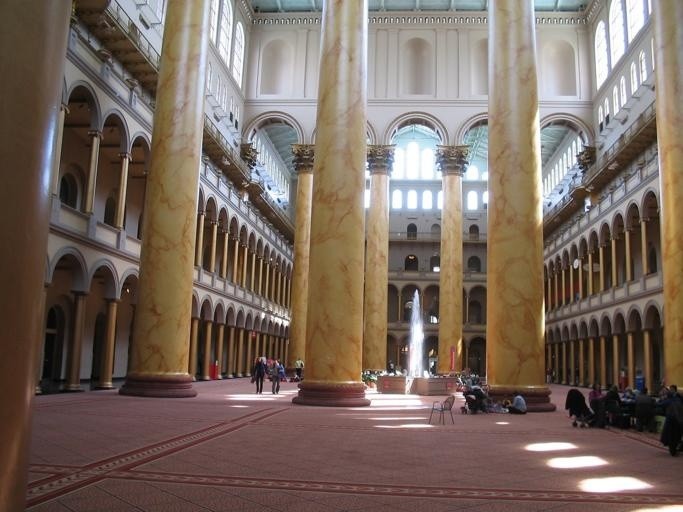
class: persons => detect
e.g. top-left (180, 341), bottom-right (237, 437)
top-left (252, 356), bottom-right (268, 394)
top-left (294, 358), bottom-right (304, 380)
top-left (506, 391), bottom-right (527, 414)
top-left (545, 368), bottom-right (552, 385)
top-left (269, 357), bottom-right (284, 393)
top-left (589, 380), bottom-right (683, 456)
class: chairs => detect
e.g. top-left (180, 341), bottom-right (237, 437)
top-left (429, 395), bottom-right (456, 426)
